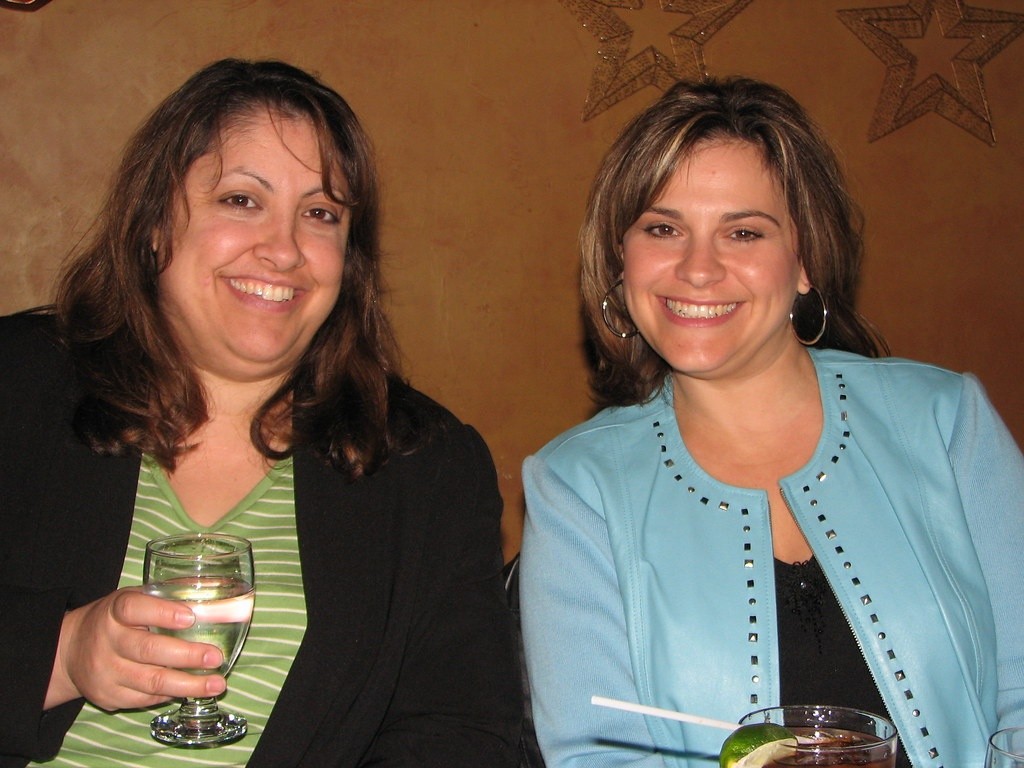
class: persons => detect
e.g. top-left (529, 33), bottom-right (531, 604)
top-left (2, 53), bottom-right (522, 768)
top-left (518, 74), bottom-right (1023, 768)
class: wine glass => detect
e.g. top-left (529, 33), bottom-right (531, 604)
top-left (143, 533), bottom-right (255, 747)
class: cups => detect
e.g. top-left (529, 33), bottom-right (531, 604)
top-left (984, 728), bottom-right (1024, 768)
top-left (739, 705), bottom-right (898, 768)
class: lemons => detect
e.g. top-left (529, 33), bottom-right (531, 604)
top-left (720, 723), bottom-right (798, 768)
